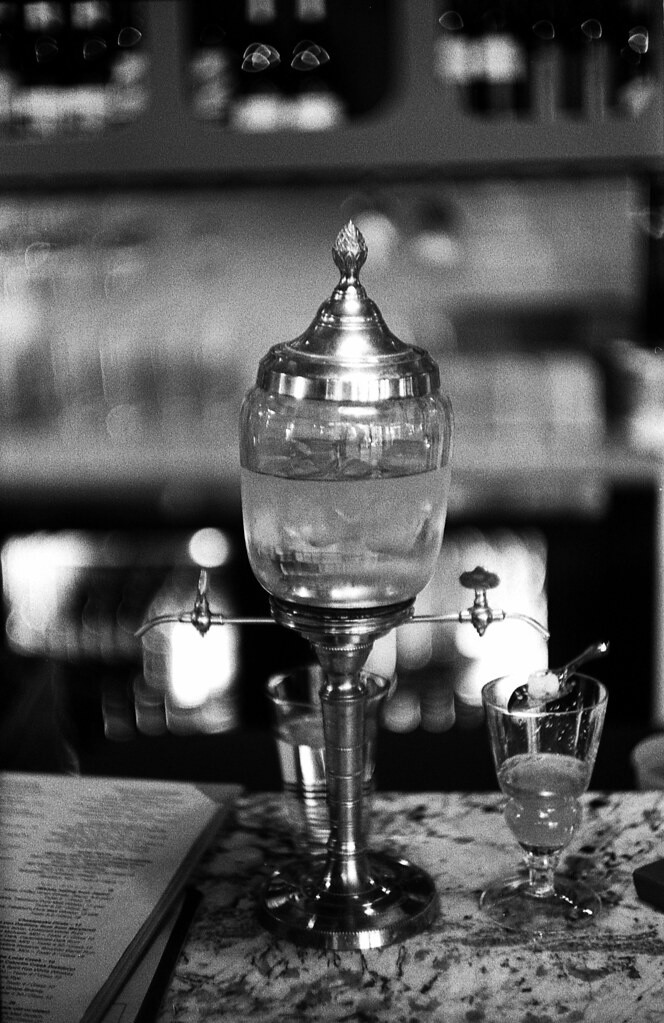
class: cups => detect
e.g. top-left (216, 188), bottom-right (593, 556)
top-left (632, 735), bottom-right (664, 858)
top-left (265, 664), bottom-right (391, 867)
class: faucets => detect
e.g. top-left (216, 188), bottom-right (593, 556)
top-left (456, 565), bottom-right (553, 641)
top-left (133, 564), bottom-right (224, 640)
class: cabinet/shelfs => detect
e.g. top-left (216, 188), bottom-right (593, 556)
top-left (1, 0), bottom-right (664, 182)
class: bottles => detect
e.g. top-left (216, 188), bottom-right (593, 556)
top-left (0, 1), bottom-right (664, 137)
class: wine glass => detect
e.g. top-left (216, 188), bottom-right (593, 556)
top-left (476, 667), bottom-right (610, 935)
